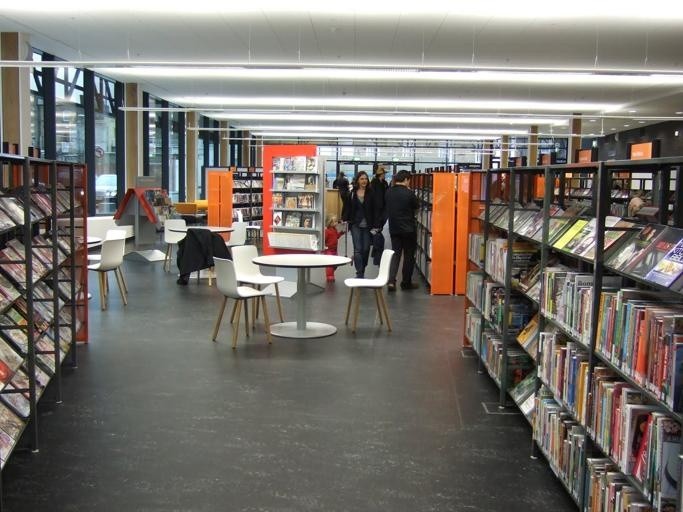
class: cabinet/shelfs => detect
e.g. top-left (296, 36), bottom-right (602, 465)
top-left (0, 148), bottom-right (89, 471)
top-left (270, 157), bottom-right (326, 248)
top-left (205, 171), bottom-right (262, 245)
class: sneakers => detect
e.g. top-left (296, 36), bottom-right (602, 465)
top-left (387, 281), bottom-right (420, 291)
top-left (371, 253), bottom-right (381, 265)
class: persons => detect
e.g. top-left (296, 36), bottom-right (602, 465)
top-left (332, 166), bottom-right (389, 267)
top-left (341, 171), bottom-right (382, 279)
top-left (319, 212), bottom-right (348, 282)
top-left (386, 169), bottom-right (420, 291)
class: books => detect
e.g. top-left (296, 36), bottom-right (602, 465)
top-left (232, 153), bottom-right (319, 238)
top-left (413, 174), bottom-right (431, 284)
top-left (462, 173), bottom-right (682, 512)
top-left (0, 180), bottom-right (82, 471)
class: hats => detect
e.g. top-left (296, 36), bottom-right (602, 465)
top-left (376, 167), bottom-right (387, 175)
